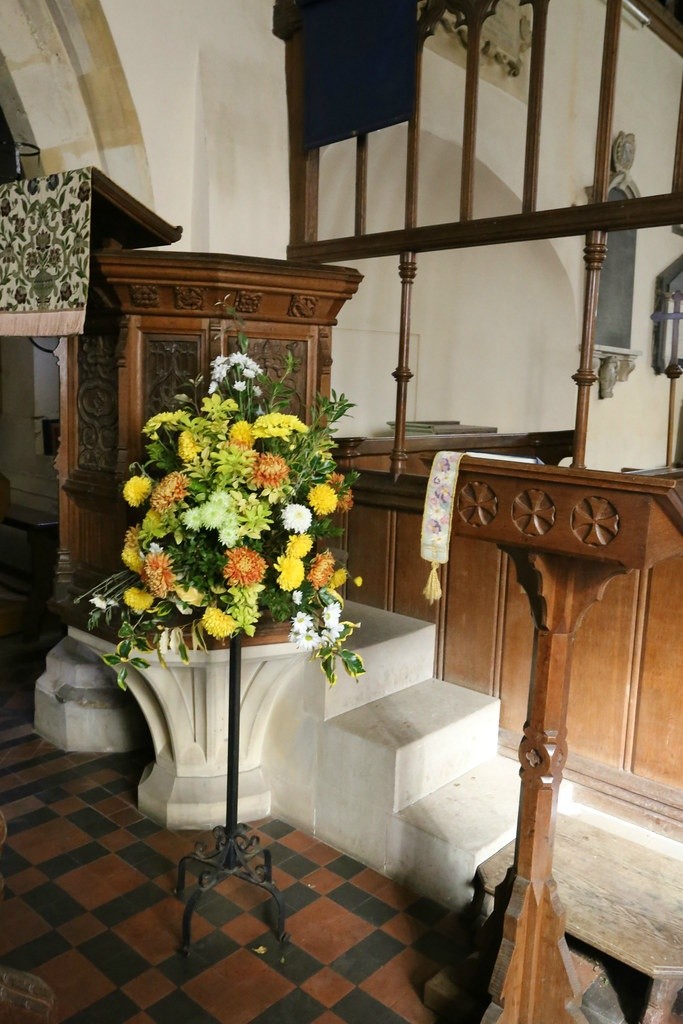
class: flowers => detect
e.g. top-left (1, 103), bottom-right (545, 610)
top-left (74, 293), bottom-right (367, 689)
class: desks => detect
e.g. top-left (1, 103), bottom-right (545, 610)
top-left (338, 429), bottom-right (578, 475)
